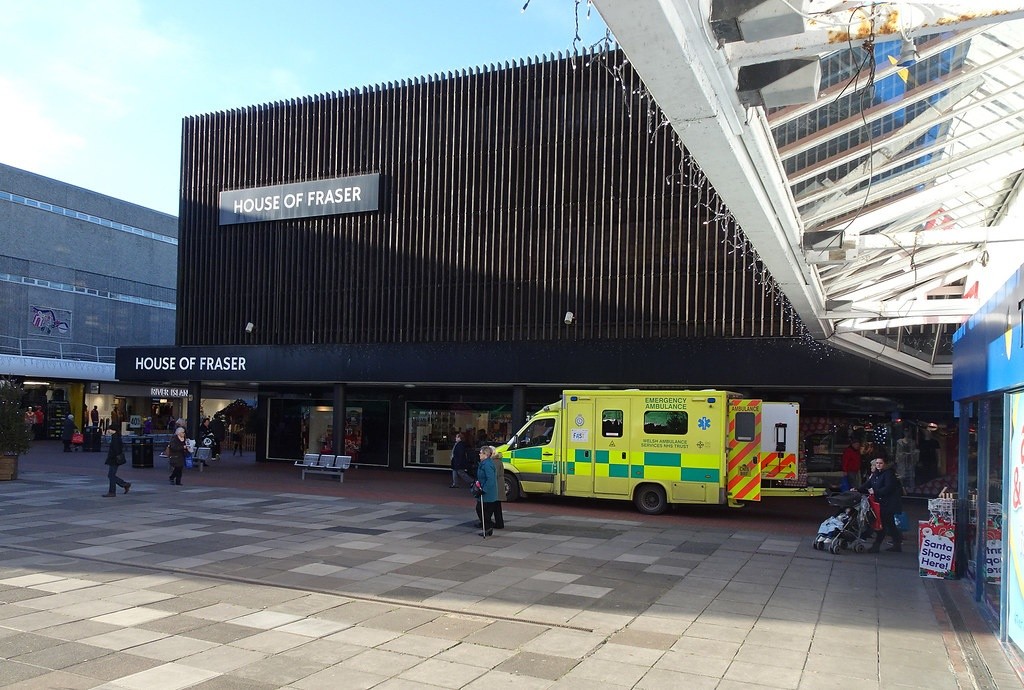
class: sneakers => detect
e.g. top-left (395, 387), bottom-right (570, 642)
top-left (817, 537), bottom-right (825, 541)
top-left (824, 538), bottom-right (832, 543)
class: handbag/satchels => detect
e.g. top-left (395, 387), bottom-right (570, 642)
top-left (869, 495), bottom-right (883, 531)
top-left (184, 451), bottom-right (193, 468)
top-left (71, 431), bottom-right (83, 444)
top-left (894, 511), bottom-right (911, 532)
top-left (115, 454), bottom-right (126, 465)
top-left (839, 476), bottom-right (849, 492)
top-left (470, 482), bottom-right (480, 497)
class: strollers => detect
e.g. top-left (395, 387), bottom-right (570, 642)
top-left (812, 491), bottom-right (872, 555)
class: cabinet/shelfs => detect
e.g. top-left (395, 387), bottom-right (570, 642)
top-left (411, 409), bottom-right (455, 463)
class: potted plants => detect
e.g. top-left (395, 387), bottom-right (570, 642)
top-left (0, 372), bottom-right (36, 481)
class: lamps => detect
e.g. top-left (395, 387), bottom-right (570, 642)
top-left (564, 312), bottom-right (576, 325)
top-left (245, 322), bottom-right (255, 333)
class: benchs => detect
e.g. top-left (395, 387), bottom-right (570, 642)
top-left (294, 454), bottom-right (352, 483)
top-left (99, 434), bottom-right (174, 452)
top-left (159, 445), bottom-right (212, 472)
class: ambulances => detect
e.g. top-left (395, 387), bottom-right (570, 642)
top-left (491, 388), bottom-right (801, 516)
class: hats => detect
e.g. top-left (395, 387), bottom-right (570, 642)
top-left (849, 435), bottom-right (861, 445)
top-left (175, 428), bottom-right (185, 434)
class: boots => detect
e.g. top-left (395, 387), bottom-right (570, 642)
top-left (169, 474), bottom-right (183, 486)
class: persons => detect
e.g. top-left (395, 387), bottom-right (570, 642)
top-left (25, 406), bottom-right (45, 441)
top-left (916, 430), bottom-right (937, 487)
top-left (100, 407), bottom-right (120, 434)
top-left (143, 417), bottom-right (152, 434)
top-left (167, 427), bottom-right (188, 485)
top-left (471, 445), bottom-right (507, 536)
top-left (199, 414), bottom-right (225, 466)
top-left (818, 436), bottom-right (903, 553)
top-left (84, 405), bottom-right (89, 427)
top-left (101, 423), bottom-right (132, 497)
top-left (62, 415), bottom-right (78, 452)
top-left (158, 416), bottom-right (187, 457)
top-left (895, 428), bottom-right (913, 495)
top-left (233, 423), bottom-right (246, 457)
top-left (90, 406), bottom-right (99, 432)
top-left (449, 427), bottom-right (494, 488)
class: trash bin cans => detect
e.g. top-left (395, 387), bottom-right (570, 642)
top-left (83, 426), bottom-right (102, 452)
top-left (131, 437), bottom-right (154, 468)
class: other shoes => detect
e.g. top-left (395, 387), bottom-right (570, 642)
top-left (102, 492), bottom-right (116, 497)
top-left (474, 521), bottom-right (503, 536)
top-left (123, 482), bottom-right (130, 494)
top-left (858, 531), bottom-right (903, 553)
